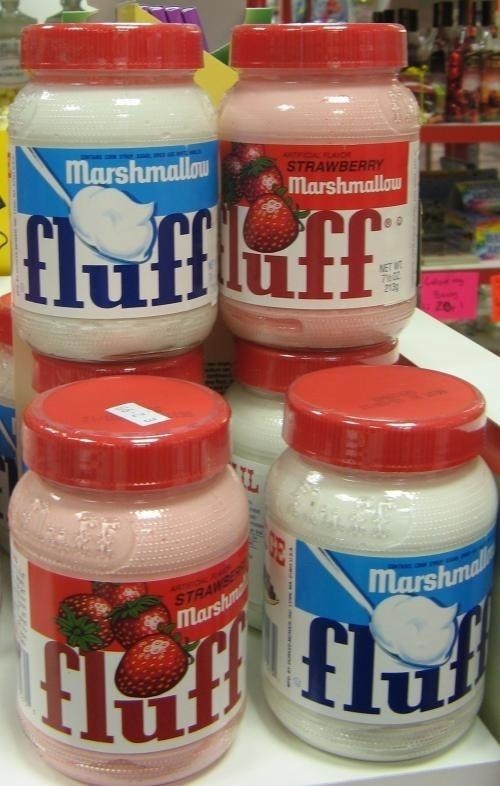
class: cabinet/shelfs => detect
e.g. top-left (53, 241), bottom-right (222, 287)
top-left (420, 122), bottom-right (500, 288)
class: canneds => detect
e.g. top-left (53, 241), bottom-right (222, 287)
top-left (262, 365), bottom-right (499, 762)
top-left (7, 373), bottom-right (249, 786)
top-left (6, 21), bottom-right (219, 362)
top-left (220, 24), bottom-right (424, 349)
top-left (2, 290), bottom-right (398, 632)
